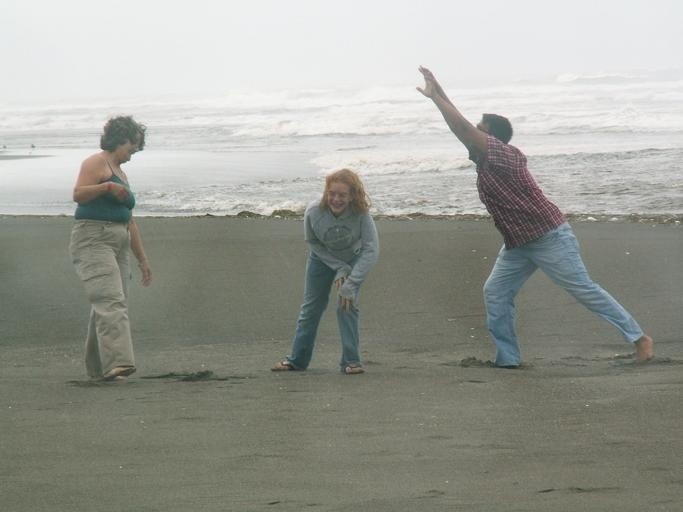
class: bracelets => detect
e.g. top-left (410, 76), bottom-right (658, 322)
top-left (107, 181), bottom-right (114, 194)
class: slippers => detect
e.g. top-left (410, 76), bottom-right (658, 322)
top-left (343, 364), bottom-right (365, 374)
top-left (271, 361), bottom-right (295, 371)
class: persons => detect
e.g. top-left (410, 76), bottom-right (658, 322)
top-left (64, 115), bottom-right (153, 383)
top-left (413, 64), bottom-right (654, 369)
top-left (267, 169), bottom-right (380, 375)
